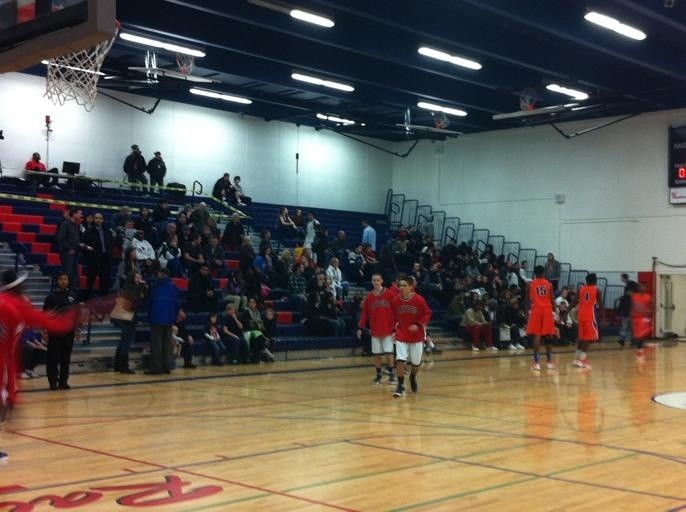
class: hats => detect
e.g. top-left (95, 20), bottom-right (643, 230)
top-left (0, 270), bottom-right (29, 292)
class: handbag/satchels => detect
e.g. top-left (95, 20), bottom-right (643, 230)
top-left (110, 297), bottom-right (135, 322)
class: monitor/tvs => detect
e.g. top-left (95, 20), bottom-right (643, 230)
top-left (62, 161), bottom-right (80, 176)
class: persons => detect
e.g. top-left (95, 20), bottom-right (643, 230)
top-left (212, 173), bottom-right (246, 206)
top-left (123, 144), bottom-right (150, 198)
top-left (26, 153), bottom-right (60, 189)
top-left (22, 323), bottom-right (48, 378)
top-left (232, 175), bottom-right (244, 195)
top-left (525, 265), bottom-right (555, 369)
top-left (223, 207), bottom-right (443, 354)
top-left (41, 274), bottom-right (74, 390)
top-left (147, 152), bottom-right (167, 197)
top-left (361, 219), bottom-right (584, 351)
top-left (0, 271), bottom-right (77, 462)
top-left (617, 273), bottom-right (641, 345)
top-left (631, 280), bottom-right (655, 361)
top-left (54, 204), bottom-right (277, 375)
top-left (564, 273), bottom-right (602, 367)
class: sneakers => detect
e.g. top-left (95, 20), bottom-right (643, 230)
top-left (361, 348), bottom-right (442, 397)
top-left (472, 340), bottom-right (656, 370)
